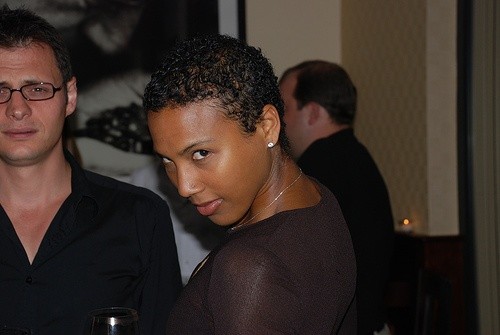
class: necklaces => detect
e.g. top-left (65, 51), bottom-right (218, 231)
top-left (231, 167), bottom-right (303, 230)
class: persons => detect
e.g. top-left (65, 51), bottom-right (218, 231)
top-left (0, 7), bottom-right (184, 335)
top-left (142, 32), bottom-right (357, 335)
top-left (278, 60), bottom-right (395, 335)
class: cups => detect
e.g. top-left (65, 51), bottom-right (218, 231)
top-left (86, 307), bottom-right (139, 335)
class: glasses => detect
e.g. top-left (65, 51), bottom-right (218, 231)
top-left (0, 82), bottom-right (63, 104)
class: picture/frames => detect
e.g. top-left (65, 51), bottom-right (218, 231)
top-left (0, 0), bottom-right (246, 287)
